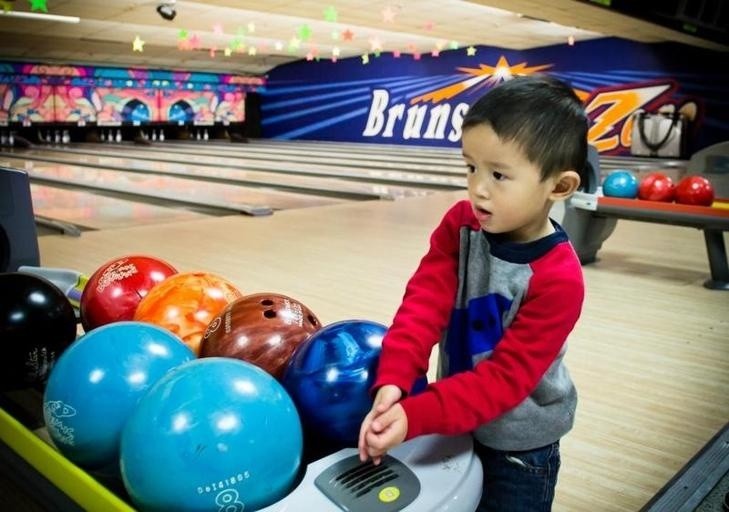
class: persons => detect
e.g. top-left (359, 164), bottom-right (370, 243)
top-left (358, 74), bottom-right (588, 512)
